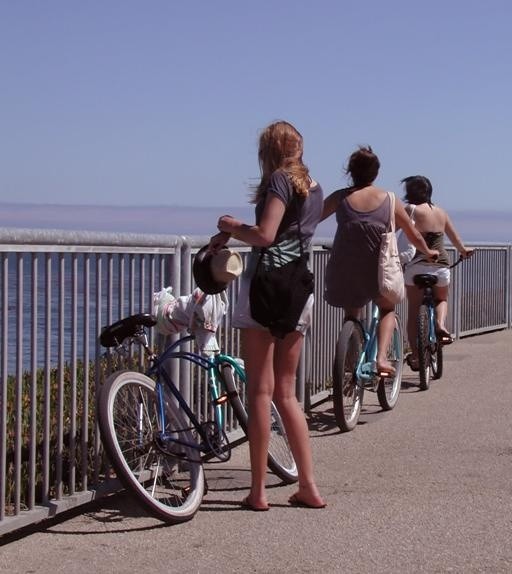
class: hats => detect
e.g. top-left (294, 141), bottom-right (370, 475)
top-left (192, 241), bottom-right (243, 296)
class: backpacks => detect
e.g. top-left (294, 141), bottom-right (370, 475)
top-left (248, 246), bottom-right (315, 339)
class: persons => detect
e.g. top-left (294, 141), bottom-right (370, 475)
top-left (206, 121), bottom-right (325, 510)
top-left (391, 174), bottom-right (473, 372)
top-left (319, 144), bottom-right (440, 398)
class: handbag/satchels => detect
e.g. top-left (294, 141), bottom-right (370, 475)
top-left (392, 203), bottom-right (417, 262)
top-left (371, 190), bottom-right (406, 303)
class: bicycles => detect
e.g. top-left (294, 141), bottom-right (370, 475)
top-left (321, 244), bottom-right (438, 432)
top-left (97, 241), bottom-right (300, 526)
top-left (406, 249), bottom-right (476, 391)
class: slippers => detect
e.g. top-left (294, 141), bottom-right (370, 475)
top-left (288, 492), bottom-right (327, 512)
top-left (241, 493), bottom-right (270, 513)
